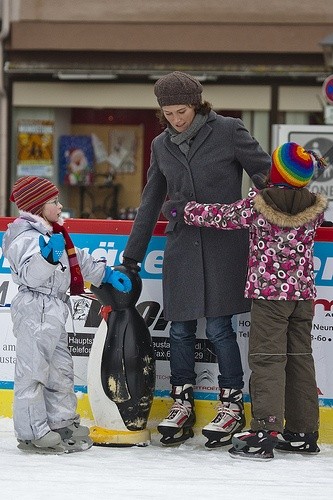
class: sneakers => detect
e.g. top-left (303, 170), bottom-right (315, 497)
top-left (53, 422), bottom-right (93, 451)
top-left (275, 428), bottom-right (320, 454)
top-left (156, 384), bottom-right (196, 446)
top-left (202, 388), bottom-right (246, 448)
top-left (228, 429), bottom-right (277, 462)
top-left (17, 430), bottom-right (65, 454)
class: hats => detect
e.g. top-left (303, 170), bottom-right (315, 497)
top-left (268, 142), bottom-right (327, 189)
top-left (9, 176), bottom-right (59, 215)
top-left (154, 71), bottom-right (203, 105)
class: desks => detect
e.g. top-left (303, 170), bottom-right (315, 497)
top-left (79, 183), bottom-right (123, 220)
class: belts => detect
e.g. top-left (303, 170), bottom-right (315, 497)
top-left (18, 284), bottom-right (76, 336)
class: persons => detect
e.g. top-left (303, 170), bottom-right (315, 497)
top-left (2, 175), bottom-right (133, 455)
top-left (121, 71), bottom-right (272, 448)
top-left (161, 143), bottom-right (330, 462)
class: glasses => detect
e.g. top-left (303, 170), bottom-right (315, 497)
top-left (45, 197), bottom-right (60, 206)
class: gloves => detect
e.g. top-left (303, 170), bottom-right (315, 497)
top-left (102, 265), bottom-right (132, 293)
top-left (161, 192), bottom-right (187, 223)
top-left (121, 256), bottom-right (141, 274)
top-left (39, 234), bottom-right (65, 265)
